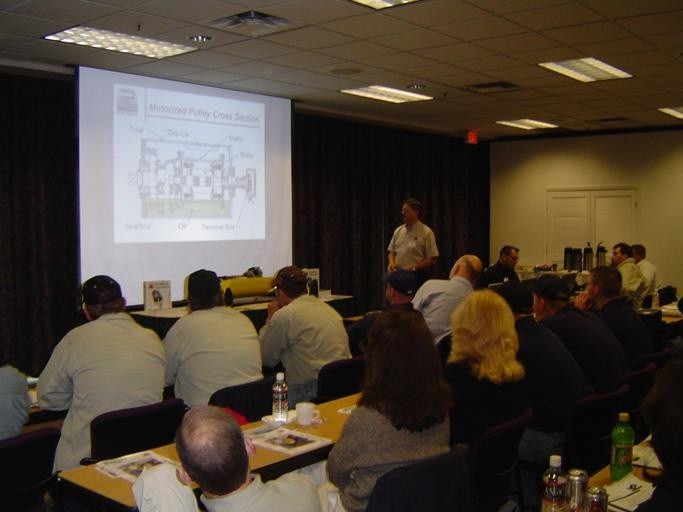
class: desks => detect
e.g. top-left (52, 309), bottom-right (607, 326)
top-left (130, 293), bottom-right (357, 340)
top-left (2, 375), bottom-right (67, 437)
top-left (59, 391), bottom-right (363, 511)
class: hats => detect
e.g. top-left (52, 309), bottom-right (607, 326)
top-left (525, 273), bottom-right (571, 302)
top-left (82, 274), bottom-right (122, 305)
top-left (380, 269), bottom-right (418, 296)
top-left (189, 269), bottom-right (220, 297)
top-left (270, 266), bottom-right (307, 287)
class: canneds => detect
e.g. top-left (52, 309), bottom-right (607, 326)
top-left (583, 485), bottom-right (607, 511)
top-left (565, 468), bottom-right (589, 511)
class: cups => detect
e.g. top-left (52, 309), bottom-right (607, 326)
top-left (296, 403), bottom-right (319, 426)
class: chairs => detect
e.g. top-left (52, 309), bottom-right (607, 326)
top-left (515, 267), bottom-right (591, 319)
top-left (633, 348), bottom-right (672, 369)
top-left (365, 439), bottom-right (508, 512)
top-left (207, 373), bottom-right (283, 422)
top-left (622, 363), bottom-right (660, 410)
top-left (1, 470), bottom-right (61, 511)
top-left (315, 358), bottom-right (365, 404)
top-left (476, 407), bottom-right (536, 510)
top-left (79, 397), bottom-right (190, 468)
top-left (566, 384), bottom-right (632, 468)
top-left (636, 285), bottom-right (682, 325)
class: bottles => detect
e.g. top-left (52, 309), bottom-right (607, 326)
top-left (653, 289), bottom-right (659, 308)
top-left (564, 246), bottom-right (608, 272)
top-left (272, 372), bottom-right (288, 420)
top-left (541, 455), bottom-right (567, 511)
top-left (610, 412), bottom-right (634, 484)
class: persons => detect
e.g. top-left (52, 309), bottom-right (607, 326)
top-left (387, 199), bottom-right (439, 285)
top-left (1, 244), bottom-right (683, 511)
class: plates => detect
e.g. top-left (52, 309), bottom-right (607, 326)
top-left (262, 414), bottom-right (293, 425)
